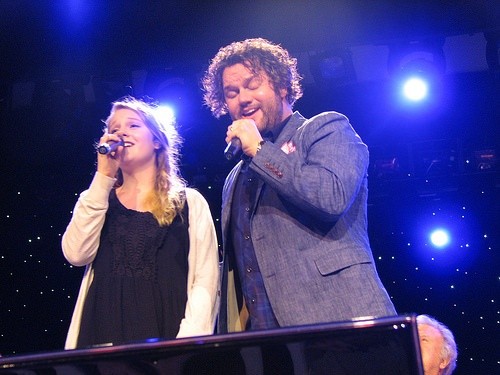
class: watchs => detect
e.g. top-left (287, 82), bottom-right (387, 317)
top-left (256, 141), bottom-right (268, 153)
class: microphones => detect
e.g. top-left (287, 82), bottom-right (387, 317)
top-left (224, 136), bottom-right (242, 160)
top-left (98, 137), bottom-right (124, 154)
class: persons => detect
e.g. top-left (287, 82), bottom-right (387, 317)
top-left (413, 315), bottom-right (458, 375)
top-left (60, 100), bottom-right (223, 355)
top-left (200, 38), bottom-right (397, 334)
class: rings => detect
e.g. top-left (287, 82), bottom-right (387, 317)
top-left (231, 126), bottom-right (233, 131)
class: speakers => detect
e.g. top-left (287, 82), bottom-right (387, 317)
top-left (0, 313), bottom-right (423, 375)
